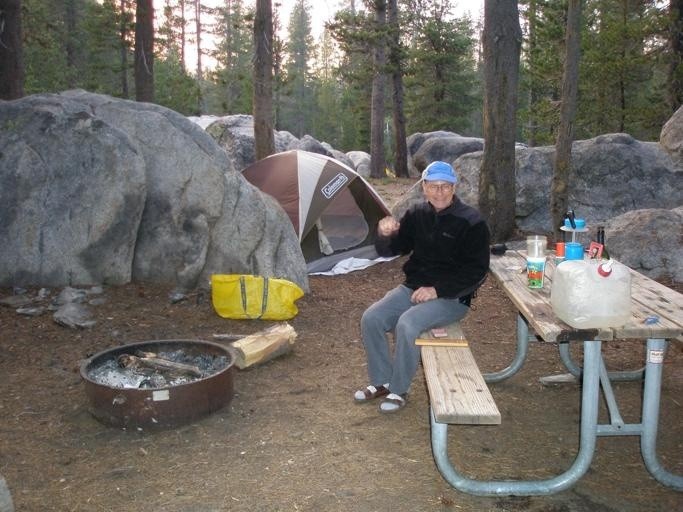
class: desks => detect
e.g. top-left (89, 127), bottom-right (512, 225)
top-left (489, 251), bottom-right (683, 494)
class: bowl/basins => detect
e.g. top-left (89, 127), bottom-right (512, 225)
top-left (78, 338), bottom-right (237, 432)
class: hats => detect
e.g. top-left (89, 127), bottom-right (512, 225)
top-left (421, 160), bottom-right (458, 183)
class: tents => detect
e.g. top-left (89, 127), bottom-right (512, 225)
top-left (240, 150), bottom-right (391, 276)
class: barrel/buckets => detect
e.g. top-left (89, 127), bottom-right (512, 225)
top-left (551, 258), bottom-right (633, 330)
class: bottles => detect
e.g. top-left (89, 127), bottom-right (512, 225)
top-left (591, 225), bottom-right (608, 260)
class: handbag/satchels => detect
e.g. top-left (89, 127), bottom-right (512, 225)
top-left (210, 273), bottom-right (305, 320)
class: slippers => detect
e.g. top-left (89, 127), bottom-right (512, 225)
top-left (354, 383), bottom-right (409, 413)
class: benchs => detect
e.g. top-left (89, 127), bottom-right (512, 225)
top-left (420, 319), bottom-right (502, 496)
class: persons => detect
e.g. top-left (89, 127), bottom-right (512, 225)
top-left (353, 160), bottom-right (491, 414)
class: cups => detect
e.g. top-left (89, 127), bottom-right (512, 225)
top-left (526, 256), bottom-right (547, 288)
top-left (564, 218), bottom-right (586, 260)
top-left (528, 235), bottom-right (547, 258)
top-left (556, 242), bottom-right (565, 257)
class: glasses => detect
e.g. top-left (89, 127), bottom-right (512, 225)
top-left (430, 184), bottom-right (449, 191)
